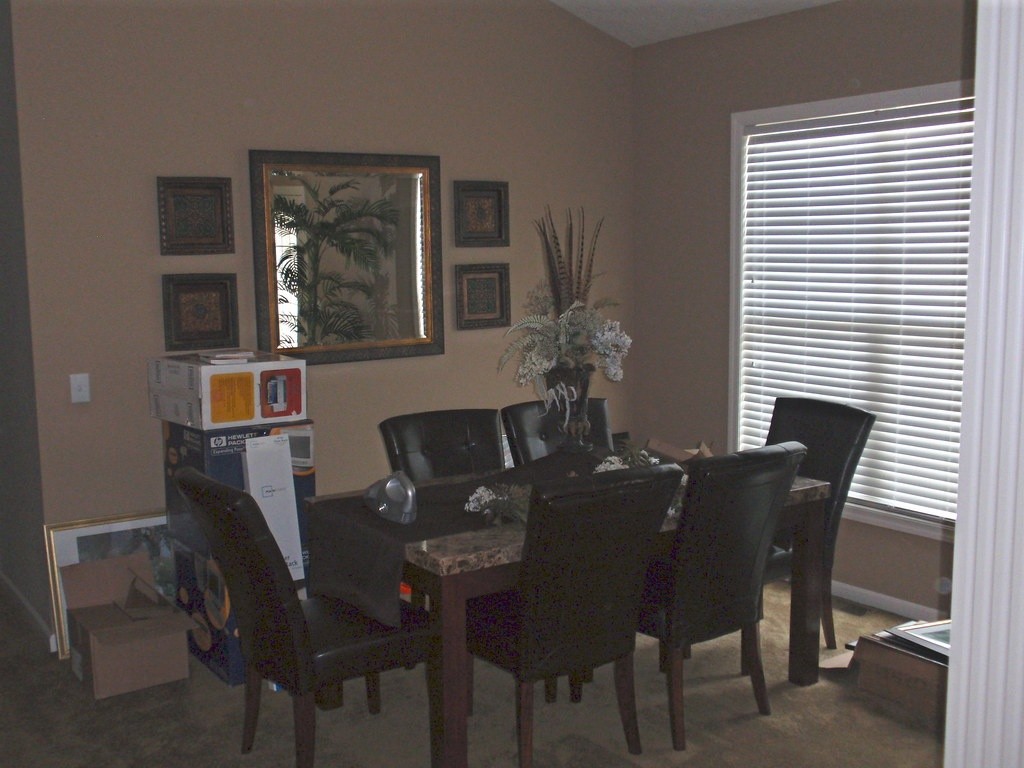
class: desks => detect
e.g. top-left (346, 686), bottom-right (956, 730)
top-left (300, 458), bottom-right (835, 768)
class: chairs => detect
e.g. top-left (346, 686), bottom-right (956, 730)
top-left (501, 391), bottom-right (613, 469)
top-left (642, 439), bottom-right (811, 751)
top-left (469, 460), bottom-right (683, 768)
top-left (744, 395), bottom-right (879, 672)
top-left (173, 462), bottom-right (430, 768)
top-left (374, 405), bottom-right (511, 480)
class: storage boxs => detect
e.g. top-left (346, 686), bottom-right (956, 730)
top-left (157, 427), bottom-right (320, 681)
top-left (148, 346), bottom-right (311, 426)
top-left (842, 620), bottom-right (947, 733)
top-left (69, 564), bottom-right (191, 701)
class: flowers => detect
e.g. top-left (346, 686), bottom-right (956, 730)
top-left (497, 198), bottom-right (636, 424)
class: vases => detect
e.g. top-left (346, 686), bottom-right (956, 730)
top-left (544, 363), bottom-right (598, 466)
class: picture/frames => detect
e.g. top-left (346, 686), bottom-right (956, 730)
top-left (153, 174), bottom-right (239, 257)
top-left (452, 178), bottom-right (512, 249)
top-left (454, 262), bottom-right (512, 332)
top-left (245, 148), bottom-right (447, 368)
top-left (161, 271), bottom-right (241, 351)
